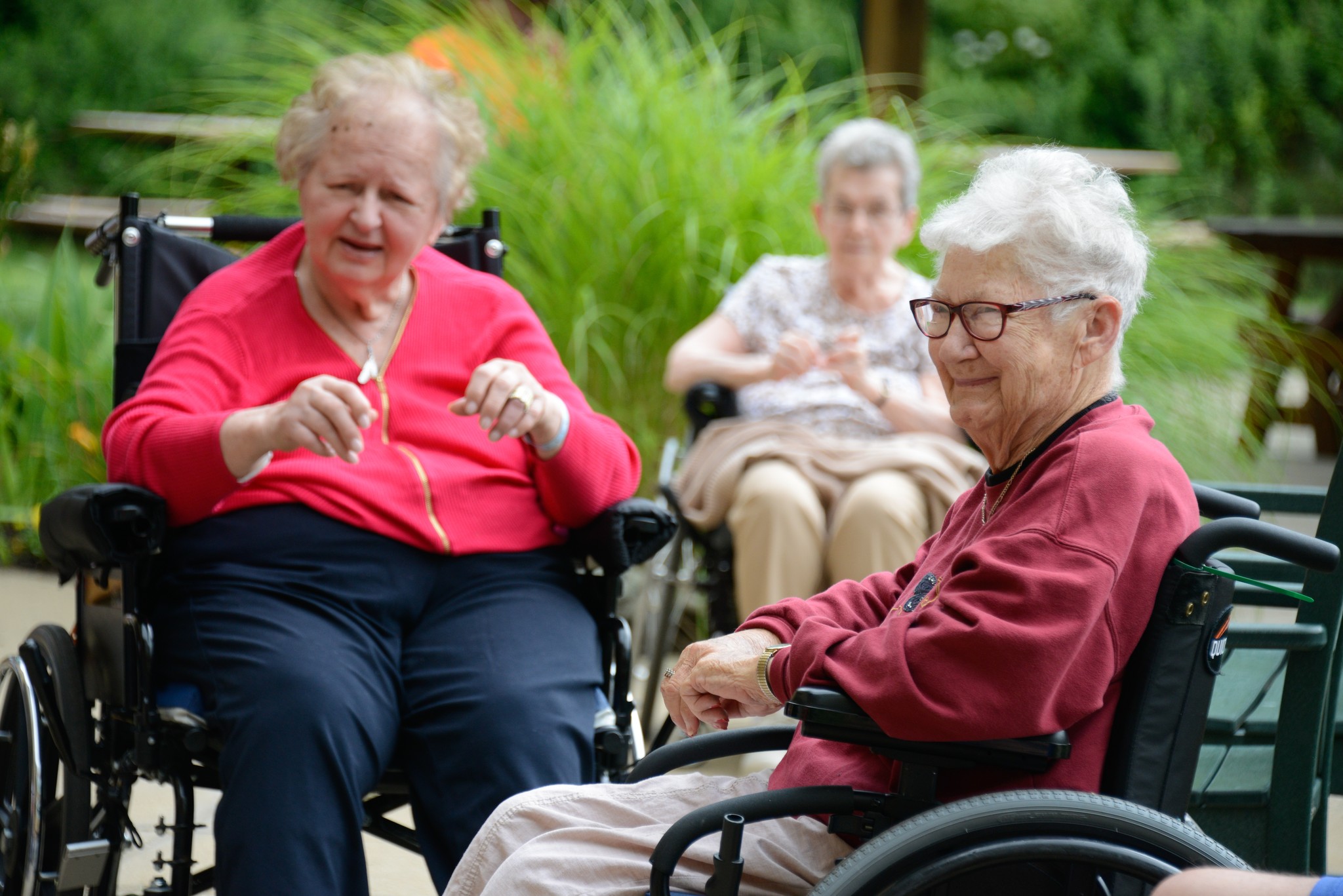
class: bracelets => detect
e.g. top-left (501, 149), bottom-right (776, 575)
top-left (523, 394), bottom-right (570, 452)
top-left (877, 383), bottom-right (892, 409)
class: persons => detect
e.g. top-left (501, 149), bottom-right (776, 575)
top-left (439, 146), bottom-right (1343, 896)
top-left (101, 52), bottom-right (644, 895)
top-left (661, 117), bottom-right (970, 623)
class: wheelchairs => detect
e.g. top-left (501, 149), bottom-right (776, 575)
top-left (622, 449), bottom-right (1343, 896)
top-left (0, 191), bottom-right (679, 896)
top-left (635, 383), bottom-right (740, 758)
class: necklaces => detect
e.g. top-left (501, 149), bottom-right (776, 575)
top-left (980, 449), bottom-right (1037, 527)
top-left (311, 274), bottom-right (403, 386)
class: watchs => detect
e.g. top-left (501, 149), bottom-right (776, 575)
top-left (756, 644), bottom-right (792, 706)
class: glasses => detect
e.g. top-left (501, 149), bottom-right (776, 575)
top-left (909, 293), bottom-right (1098, 339)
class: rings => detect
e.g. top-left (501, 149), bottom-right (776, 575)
top-left (664, 669), bottom-right (675, 679)
top-left (510, 384), bottom-right (534, 412)
